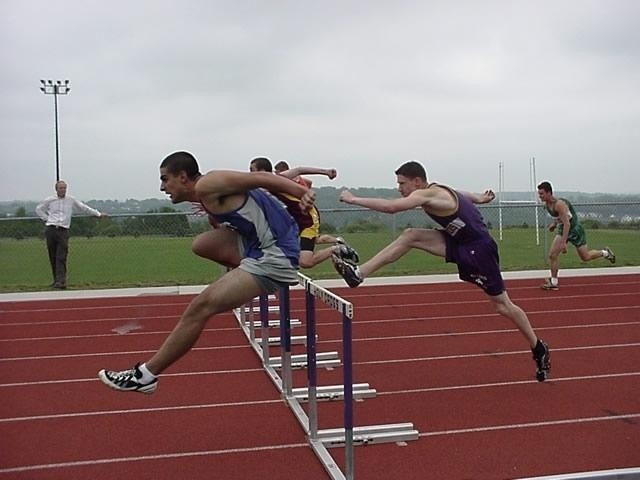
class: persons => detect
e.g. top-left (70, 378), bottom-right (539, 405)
top-left (330, 160), bottom-right (552, 383)
top-left (537, 181), bottom-right (616, 291)
top-left (99, 152), bottom-right (315, 394)
top-left (191, 158), bottom-right (359, 268)
top-left (275, 160), bottom-right (348, 245)
top-left (34, 180), bottom-right (107, 289)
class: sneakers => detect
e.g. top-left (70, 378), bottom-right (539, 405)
top-left (97, 364), bottom-right (157, 395)
top-left (532, 341), bottom-right (550, 383)
top-left (331, 252), bottom-right (363, 289)
top-left (541, 281), bottom-right (559, 291)
top-left (335, 236), bottom-right (359, 264)
top-left (603, 246), bottom-right (616, 264)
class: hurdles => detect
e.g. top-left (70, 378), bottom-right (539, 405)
top-left (233, 273), bottom-right (419, 480)
